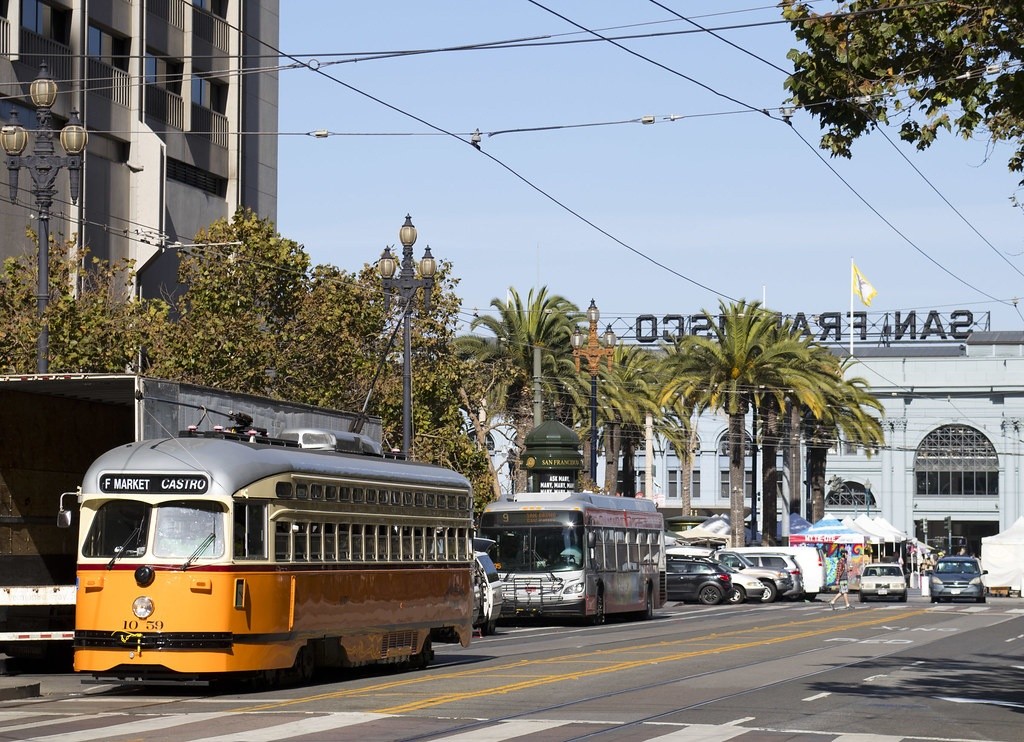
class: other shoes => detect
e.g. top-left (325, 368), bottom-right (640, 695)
top-left (847, 605), bottom-right (855, 610)
top-left (829, 602), bottom-right (835, 609)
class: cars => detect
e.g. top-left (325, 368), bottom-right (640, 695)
top-left (743, 552), bottom-right (805, 601)
top-left (856, 561), bottom-right (908, 603)
top-left (472, 549), bottom-right (503, 634)
top-left (703, 558), bottom-right (765, 605)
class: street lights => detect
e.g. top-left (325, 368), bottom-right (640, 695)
top-left (570, 296), bottom-right (618, 489)
top-left (863, 478), bottom-right (873, 517)
top-left (378, 216), bottom-right (437, 459)
top-left (2, 58), bottom-right (87, 373)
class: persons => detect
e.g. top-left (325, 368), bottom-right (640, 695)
top-left (828, 550), bottom-right (856, 610)
top-left (956, 547), bottom-right (971, 557)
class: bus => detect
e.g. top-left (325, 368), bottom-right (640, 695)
top-left (58, 412), bottom-right (474, 692)
top-left (479, 491), bottom-right (669, 626)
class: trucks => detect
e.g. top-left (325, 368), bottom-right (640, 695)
top-left (715, 546), bottom-right (827, 601)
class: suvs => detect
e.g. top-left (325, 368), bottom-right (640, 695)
top-left (711, 551), bottom-right (794, 603)
top-left (664, 555), bottom-right (736, 606)
top-left (926, 555), bottom-right (989, 604)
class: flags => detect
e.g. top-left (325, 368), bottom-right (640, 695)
top-left (851, 263), bottom-right (877, 306)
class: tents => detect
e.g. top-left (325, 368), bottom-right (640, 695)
top-left (685, 510), bottom-right (933, 588)
top-left (980, 515), bottom-right (1023, 597)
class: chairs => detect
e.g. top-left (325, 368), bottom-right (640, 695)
top-left (869, 570), bottom-right (877, 576)
top-left (888, 568), bottom-right (896, 576)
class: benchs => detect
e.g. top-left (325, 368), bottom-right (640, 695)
top-left (245, 533), bottom-right (466, 561)
